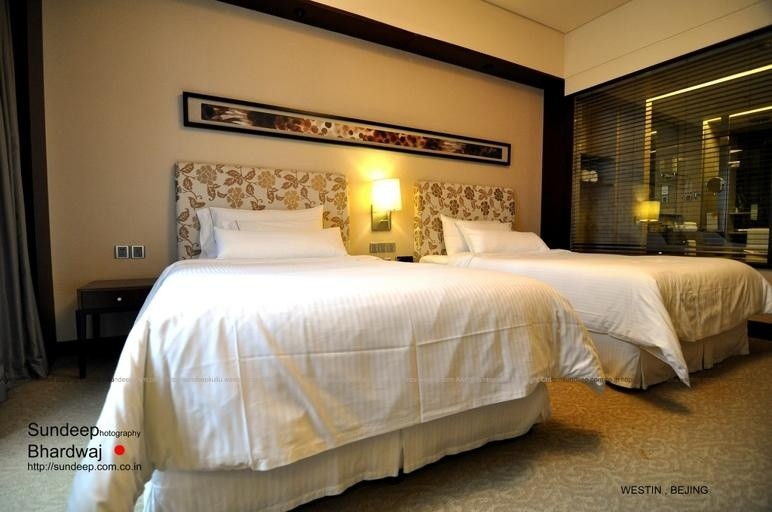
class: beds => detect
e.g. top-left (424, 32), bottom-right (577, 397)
top-left (144, 161), bottom-right (749, 512)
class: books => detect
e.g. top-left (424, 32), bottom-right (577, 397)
top-left (743, 228), bottom-right (769, 263)
top-left (706, 213), bottom-right (719, 232)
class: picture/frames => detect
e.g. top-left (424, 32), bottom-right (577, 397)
top-left (183, 90), bottom-right (510, 166)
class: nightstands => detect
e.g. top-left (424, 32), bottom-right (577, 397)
top-left (75, 279), bottom-right (157, 379)
